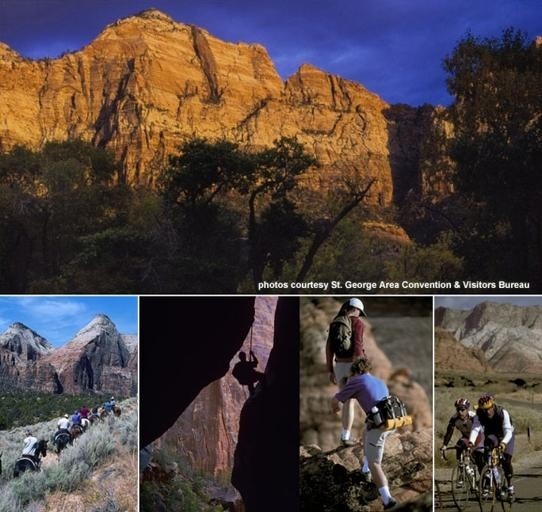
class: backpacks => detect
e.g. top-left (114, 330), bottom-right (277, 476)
top-left (330, 315), bottom-right (358, 357)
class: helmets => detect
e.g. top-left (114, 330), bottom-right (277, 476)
top-left (478, 394), bottom-right (496, 410)
top-left (455, 398), bottom-right (470, 408)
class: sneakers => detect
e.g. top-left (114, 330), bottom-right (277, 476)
top-left (356, 470), bottom-right (371, 480)
top-left (381, 497), bottom-right (396, 510)
top-left (508, 486), bottom-right (514, 496)
top-left (339, 437), bottom-right (356, 445)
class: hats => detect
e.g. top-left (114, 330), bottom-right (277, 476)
top-left (350, 298), bottom-right (367, 318)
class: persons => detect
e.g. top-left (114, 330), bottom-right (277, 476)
top-left (440, 397), bottom-right (492, 493)
top-left (71, 394), bottom-right (116, 434)
top-left (467, 395), bottom-right (516, 495)
top-left (232, 350), bottom-right (263, 395)
top-left (52, 413), bottom-right (73, 446)
top-left (326, 298), bottom-right (368, 448)
top-left (331, 357), bottom-right (398, 511)
top-left (18, 430), bottom-right (40, 468)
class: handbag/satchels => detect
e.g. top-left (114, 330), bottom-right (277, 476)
top-left (370, 397), bottom-right (412, 429)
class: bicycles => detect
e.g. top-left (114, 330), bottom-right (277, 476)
top-left (440, 442), bottom-right (516, 512)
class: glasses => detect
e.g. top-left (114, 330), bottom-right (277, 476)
top-left (456, 408), bottom-right (465, 412)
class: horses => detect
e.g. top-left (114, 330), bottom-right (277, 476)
top-left (13, 438), bottom-right (50, 478)
top-left (54, 404), bottom-right (122, 453)
